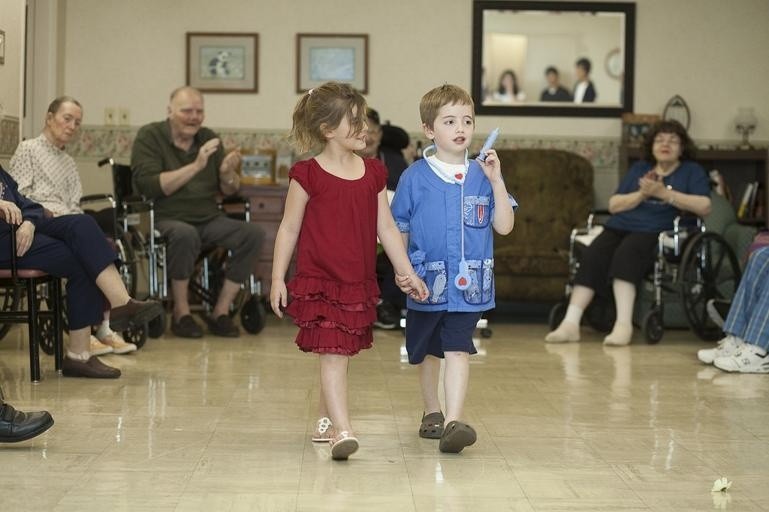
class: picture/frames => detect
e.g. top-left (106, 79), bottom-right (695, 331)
top-left (621, 113), bottom-right (658, 146)
top-left (296, 33), bottom-right (369, 94)
top-left (227, 147), bottom-right (276, 185)
top-left (185, 31), bottom-right (258, 93)
top-left (0, 30), bottom-right (5, 63)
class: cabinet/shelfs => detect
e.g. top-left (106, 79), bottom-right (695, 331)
top-left (217, 183), bottom-right (295, 304)
top-left (616, 142), bottom-right (768, 232)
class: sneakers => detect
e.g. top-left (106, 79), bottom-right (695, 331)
top-left (371, 305), bottom-right (396, 329)
top-left (696, 337), bottom-right (741, 364)
top-left (713, 348), bottom-right (769, 374)
top-left (64, 335), bottom-right (113, 356)
top-left (94, 333), bottom-right (137, 354)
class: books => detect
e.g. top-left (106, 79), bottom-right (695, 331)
top-left (736, 182), bottom-right (765, 218)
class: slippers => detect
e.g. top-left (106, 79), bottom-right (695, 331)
top-left (330, 431), bottom-right (360, 461)
top-left (311, 416), bottom-right (336, 442)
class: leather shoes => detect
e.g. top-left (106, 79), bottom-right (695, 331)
top-left (170, 314), bottom-right (204, 339)
top-left (108, 298), bottom-right (162, 333)
top-left (0, 402), bottom-right (55, 443)
top-left (61, 354), bottom-right (121, 379)
top-left (206, 314), bottom-right (240, 337)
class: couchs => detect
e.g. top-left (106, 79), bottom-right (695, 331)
top-left (632, 191), bottom-right (758, 329)
top-left (467, 147), bottom-right (595, 319)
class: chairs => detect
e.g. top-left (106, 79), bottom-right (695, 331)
top-left (0, 208), bottom-right (65, 384)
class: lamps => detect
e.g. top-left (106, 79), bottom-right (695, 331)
top-left (734, 107), bottom-right (757, 150)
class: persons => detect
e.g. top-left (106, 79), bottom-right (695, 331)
top-left (0, 383), bottom-right (55, 443)
top-left (494, 69), bottom-right (524, 103)
top-left (541, 66), bottom-right (571, 101)
top-left (387, 80), bottom-right (521, 454)
top-left (571, 58), bottom-right (597, 102)
top-left (265, 81), bottom-right (431, 461)
top-left (696, 232), bottom-right (769, 375)
top-left (0, 152), bottom-right (163, 379)
top-left (542, 118), bottom-right (713, 348)
top-left (6, 95), bottom-right (137, 357)
top-left (128, 85), bottom-right (266, 340)
top-left (354, 104), bottom-right (412, 331)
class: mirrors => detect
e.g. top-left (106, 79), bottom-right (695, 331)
top-left (470, 0), bottom-right (636, 118)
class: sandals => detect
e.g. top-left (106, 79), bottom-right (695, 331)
top-left (439, 421), bottom-right (477, 453)
top-left (418, 410), bottom-right (446, 439)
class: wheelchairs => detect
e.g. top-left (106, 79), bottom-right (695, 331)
top-left (97, 155), bottom-right (265, 340)
top-left (549, 182), bottom-right (741, 345)
top-left (37, 192), bottom-right (150, 356)
